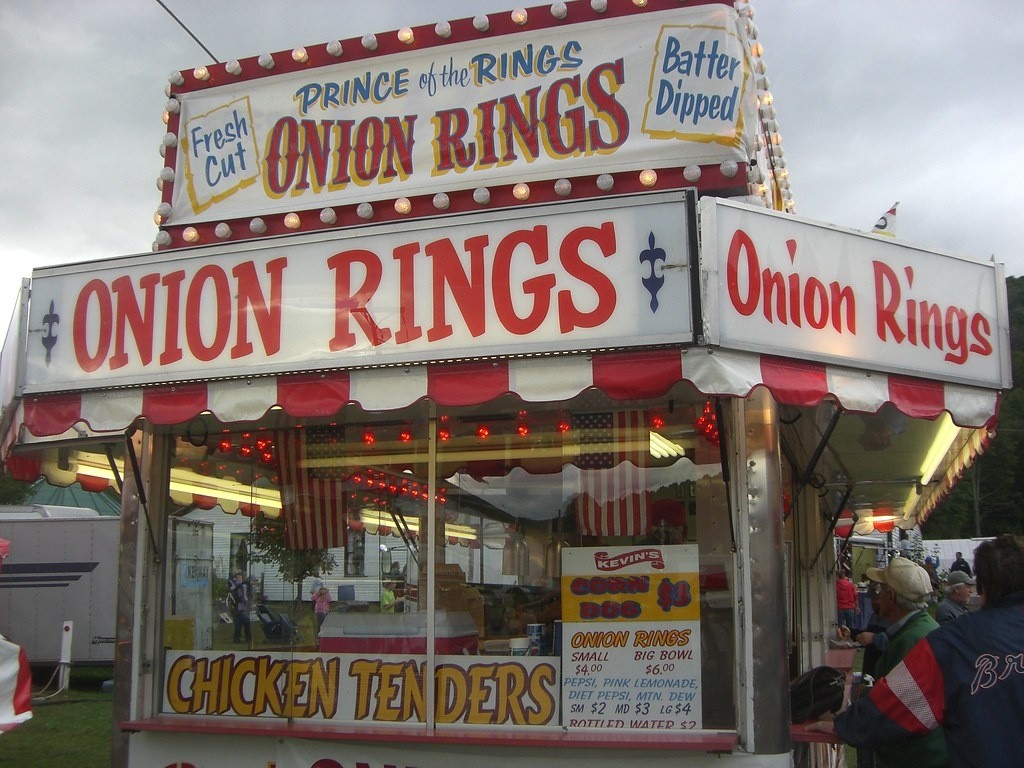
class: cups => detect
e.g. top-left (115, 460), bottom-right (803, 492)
top-left (850, 671), bottom-right (862, 705)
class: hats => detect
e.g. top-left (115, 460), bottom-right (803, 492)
top-left (866, 557), bottom-right (933, 602)
top-left (925, 557), bottom-right (933, 562)
top-left (246, 575), bottom-right (259, 582)
top-left (234, 568), bottom-right (242, 576)
top-left (947, 569), bottom-right (977, 586)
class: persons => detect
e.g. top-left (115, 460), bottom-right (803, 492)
top-left (835, 549), bottom-right (976, 768)
top-left (808, 533), bottom-right (1024, 768)
top-left (226, 561), bottom-right (408, 643)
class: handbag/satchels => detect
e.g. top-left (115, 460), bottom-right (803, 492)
top-left (226, 584), bottom-right (241, 613)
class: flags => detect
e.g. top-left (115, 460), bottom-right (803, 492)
top-left (570, 409), bottom-right (650, 537)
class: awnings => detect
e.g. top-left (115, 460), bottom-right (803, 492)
top-left (2, 189), bottom-right (1024, 550)
